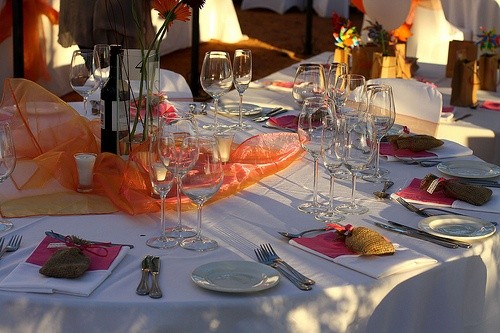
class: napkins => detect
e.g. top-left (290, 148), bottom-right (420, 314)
top-left (389, 178), bottom-right (500, 213)
top-left (130, 96), bottom-right (180, 122)
top-left (262, 114), bottom-right (322, 133)
top-left (366, 137), bottom-right (473, 162)
top-left (0, 234), bottom-right (130, 297)
top-left (288, 230), bottom-right (439, 280)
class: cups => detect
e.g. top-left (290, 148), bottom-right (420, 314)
top-left (74, 152), bottom-right (97, 193)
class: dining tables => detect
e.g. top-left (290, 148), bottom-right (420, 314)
top-left (0, 51), bottom-right (500, 333)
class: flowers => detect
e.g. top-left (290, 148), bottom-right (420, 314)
top-left (125, 0), bottom-right (204, 143)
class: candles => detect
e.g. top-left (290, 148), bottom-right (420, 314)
top-left (216, 134), bottom-right (232, 163)
top-left (75, 153), bottom-right (96, 186)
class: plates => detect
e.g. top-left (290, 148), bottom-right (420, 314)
top-left (209, 102), bottom-right (263, 116)
top-left (191, 260), bottom-right (281, 293)
top-left (417, 215), bottom-right (497, 240)
top-left (436, 159), bottom-right (500, 178)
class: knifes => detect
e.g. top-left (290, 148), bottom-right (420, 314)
top-left (251, 107), bottom-right (288, 123)
top-left (374, 221), bottom-right (472, 249)
top-left (135, 254), bottom-right (163, 298)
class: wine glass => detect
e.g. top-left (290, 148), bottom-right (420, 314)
top-left (200, 51), bottom-right (233, 130)
top-left (175, 136), bottom-right (225, 252)
top-left (0, 120), bottom-right (17, 235)
top-left (146, 135), bottom-right (178, 249)
top-left (291, 63), bottom-right (396, 223)
top-left (158, 114), bottom-right (200, 238)
top-left (69, 49), bottom-right (102, 119)
top-left (92, 45), bottom-right (119, 137)
top-left (229, 49), bottom-right (254, 130)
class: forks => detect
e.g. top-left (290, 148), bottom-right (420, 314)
top-left (396, 197), bottom-right (497, 226)
top-left (0, 234), bottom-right (23, 260)
top-left (254, 242), bottom-right (315, 291)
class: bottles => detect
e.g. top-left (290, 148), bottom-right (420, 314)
top-left (99, 49), bottom-right (131, 156)
